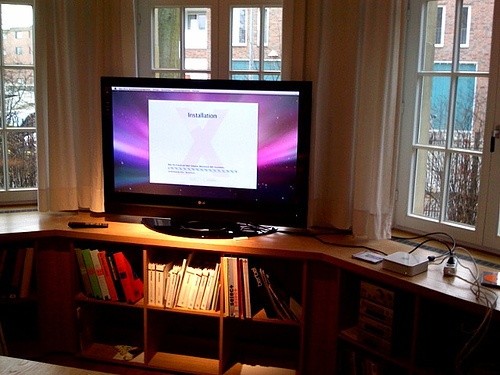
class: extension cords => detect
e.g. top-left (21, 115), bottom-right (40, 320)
top-left (444, 255), bottom-right (457, 276)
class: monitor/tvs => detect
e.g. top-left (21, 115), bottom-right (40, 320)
top-left (100, 76), bottom-right (313, 229)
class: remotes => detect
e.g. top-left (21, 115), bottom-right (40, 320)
top-left (68, 221), bottom-right (108, 228)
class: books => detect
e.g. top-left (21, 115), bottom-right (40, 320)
top-left (11, 242), bottom-right (303, 323)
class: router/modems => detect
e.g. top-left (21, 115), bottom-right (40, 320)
top-left (383, 251), bottom-right (428, 276)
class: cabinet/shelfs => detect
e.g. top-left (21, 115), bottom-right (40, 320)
top-left (1, 206), bottom-right (500, 375)
